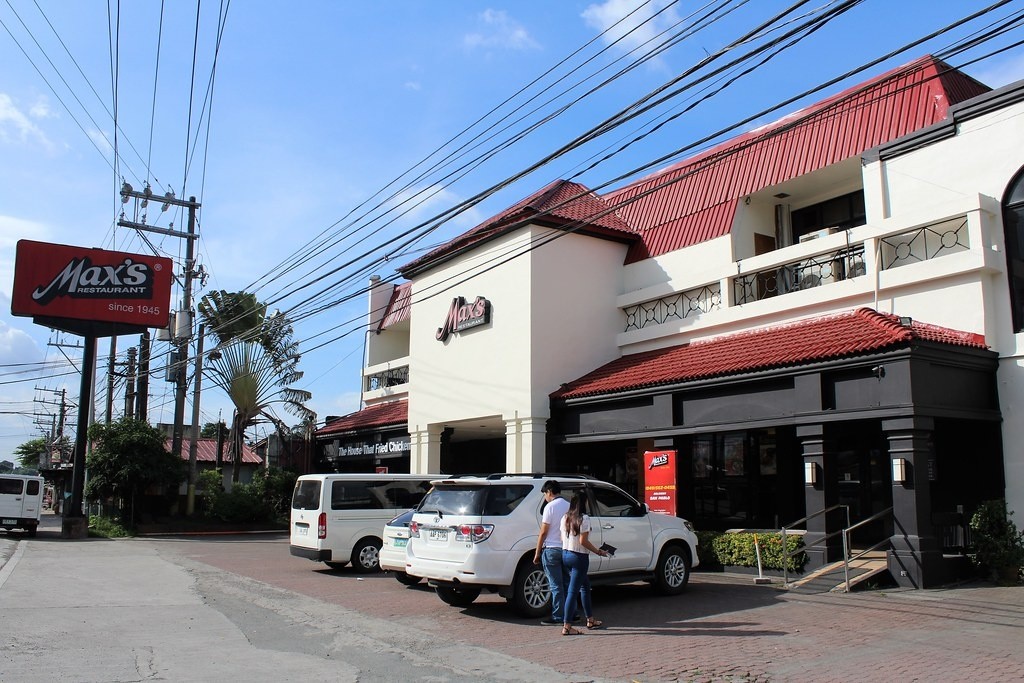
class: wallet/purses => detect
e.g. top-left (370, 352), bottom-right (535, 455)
top-left (599, 542), bottom-right (617, 556)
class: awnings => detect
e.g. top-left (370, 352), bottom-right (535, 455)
top-left (37, 467), bottom-right (74, 486)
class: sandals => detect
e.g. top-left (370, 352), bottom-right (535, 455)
top-left (561, 626), bottom-right (582, 635)
top-left (586, 617), bottom-right (603, 628)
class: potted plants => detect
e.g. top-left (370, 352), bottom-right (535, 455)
top-left (965, 500), bottom-right (1024, 587)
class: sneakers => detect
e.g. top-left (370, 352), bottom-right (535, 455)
top-left (540, 618), bottom-right (564, 627)
top-left (571, 614), bottom-right (582, 624)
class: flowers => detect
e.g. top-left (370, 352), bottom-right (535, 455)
top-left (693, 530), bottom-right (808, 571)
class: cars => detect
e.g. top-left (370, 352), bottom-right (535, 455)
top-left (378, 505), bottom-right (424, 584)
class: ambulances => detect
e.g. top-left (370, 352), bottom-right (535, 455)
top-left (0, 474), bottom-right (44, 537)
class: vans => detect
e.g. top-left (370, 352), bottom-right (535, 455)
top-left (289, 474), bottom-right (478, 574)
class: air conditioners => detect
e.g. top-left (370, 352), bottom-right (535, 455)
top-left (798, 227), bottom-right (838, 288)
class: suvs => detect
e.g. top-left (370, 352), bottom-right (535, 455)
top-left (405, 473), bottom-right (700, 618)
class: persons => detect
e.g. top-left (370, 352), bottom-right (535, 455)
top-left (624, 447), bottom-right (639, 499)
top-left (560, 492), bottom-right (609, 635)
top-left (534, 481), bottom-right (583, 626)
top-left (693, 456), bottom-right (709, 477)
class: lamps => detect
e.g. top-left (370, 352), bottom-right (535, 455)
top-left (893, 458), bottom-right (906, 482)
top-left (899, 316), bottom-right (912, 326)
top-left (804, 462), bottom-right (817, 486)
